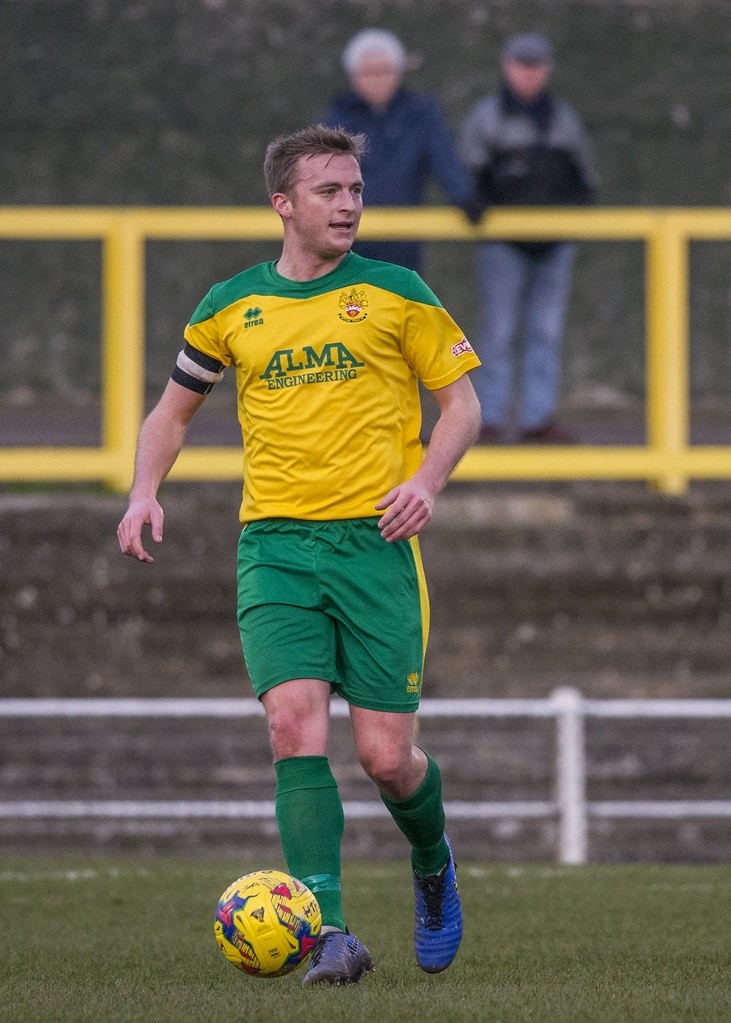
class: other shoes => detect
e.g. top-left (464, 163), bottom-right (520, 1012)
top-left (521, 427), bottom-right (579, 447)
top-left (476, 422), bottom-right (502, 444)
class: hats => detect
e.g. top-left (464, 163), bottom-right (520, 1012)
top-left (506, 30), bottom-right (552, 66)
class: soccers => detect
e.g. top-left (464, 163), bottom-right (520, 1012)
top-left (212, 868), bottom-right (324, 980)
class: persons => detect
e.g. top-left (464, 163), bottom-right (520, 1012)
top-left (457, 34), bottom-right (607, 444)
top-left (320, 24), bottom-right (486, 276)
top-left (116, 122), bottom-right (480, 982)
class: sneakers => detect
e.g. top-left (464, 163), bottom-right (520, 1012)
top-left (411, 834), bottom-right (464, 974)
top-left (301, 925), bottom-right (375, 987)
